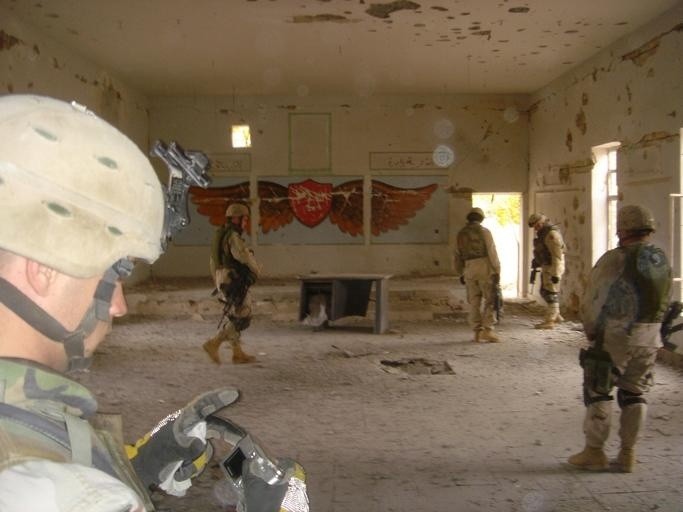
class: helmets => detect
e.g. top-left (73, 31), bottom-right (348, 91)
top-left (225, 203), bottom-right (249, 217)
top-left (528, 213), bottom-right (544, 228)
top-left (617, 205), bottom-right (656, 231)
top-left (0, 94), bottom-right (164, 276)
top-left (466, 207), bottom-right (484, 218)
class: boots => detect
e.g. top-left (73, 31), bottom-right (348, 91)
top-left (556, 315), bottom-right (564, 322)
top-left (203, 337), bottom-right (222, 362)
top-left (475, 328), bottom-right (502, 342)
top-left (617, 449), bottom-right (635, 473)
top-left (535, 319), bottom-right (554, 329)
top-left (232, 343), bottom-right (255, 362)
top-left (567, 444), bottom-right (610, 471)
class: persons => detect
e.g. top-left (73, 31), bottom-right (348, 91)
top-left (566, 203), bottom-right (673, 473)
top-left (454, 208), bottom-right (501, 344)
top-left (528, 213), bottom-right (567, 331)
top-left (0, 92), bottom-right (311, 512)
top-left (201, 203), bottom-right (261, 364)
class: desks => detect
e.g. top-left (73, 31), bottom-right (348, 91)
top-left (294, 271), bottom-right (394, 336)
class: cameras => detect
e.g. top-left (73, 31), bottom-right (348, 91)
top-left (220, 433), bottom-right (285, 500)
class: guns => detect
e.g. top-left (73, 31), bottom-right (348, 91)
top-left (529, 227), bottom-right (547, 284)
top-left (660, 300), bottom-right (682, 352)
top-left (217, 262), bottom-right (257, 330)
top-left (578, 348), bottom-right (597, 391)
top-left (492, 273), bottom-right (504, 321)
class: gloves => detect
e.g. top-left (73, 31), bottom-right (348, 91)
top-left (215, 453), bottom-right (310, 512)
top-left (122, 388), bottom-right (239, 501)
